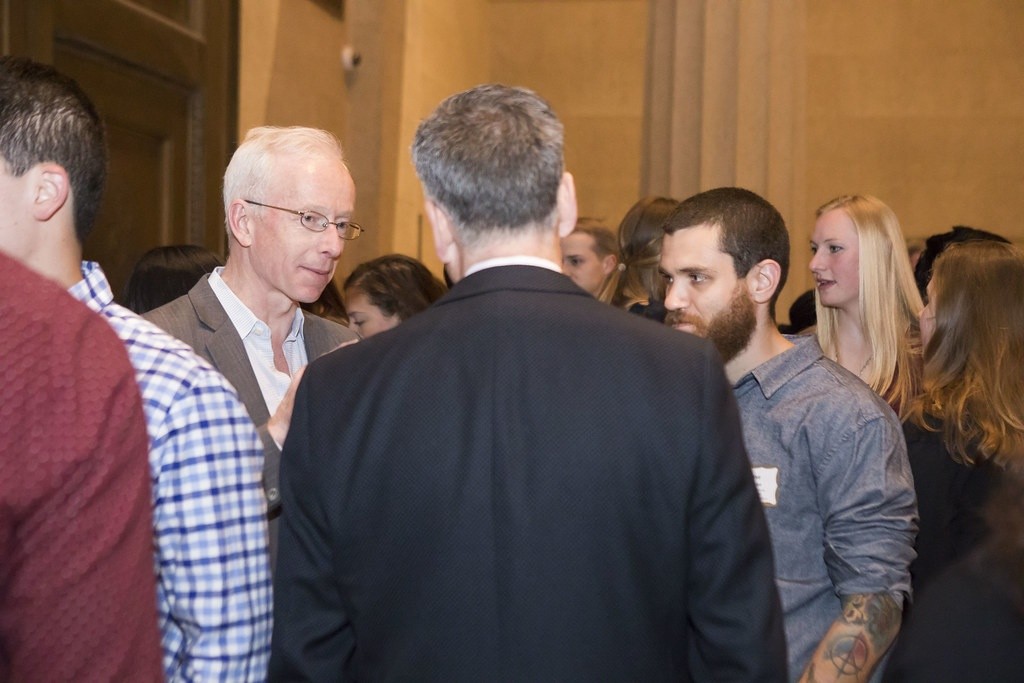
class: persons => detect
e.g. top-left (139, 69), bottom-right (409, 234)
top-left (126, 245), bottom-right (226, 317)
top-left (0, 56), bottom-right (274, 683)
top-left (342, 253), bottom-right (447, 339)
top-left (661, 187), bottom-right (921, 683)
top-left (0, 248), bottom-right (163, 683)
top-left (563, 195), bottom-right (680, 323)
top-left (791, 195), bottom-right (1024, 683)
top-left (139, 125), bottom-right (363, 494)
top-left (271, 84), bottom-right (789, 683)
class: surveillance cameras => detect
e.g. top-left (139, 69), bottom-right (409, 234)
top-left (342, 45), bottom-right (361, 71)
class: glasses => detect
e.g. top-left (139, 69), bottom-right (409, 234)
top-left (244, 200), bottom-right (364, 241)
